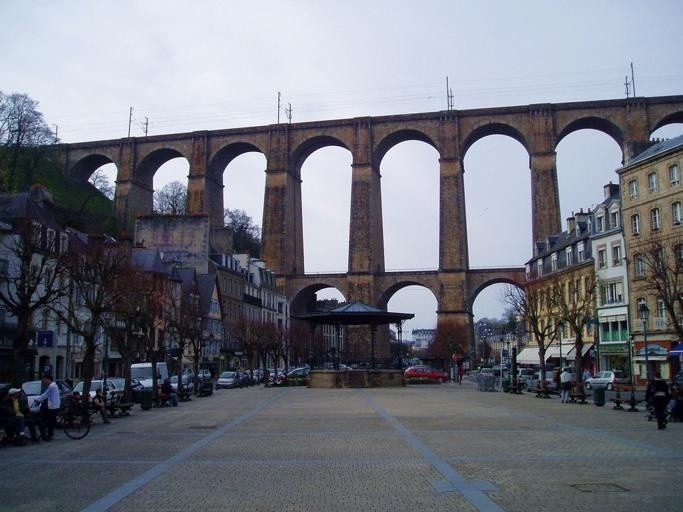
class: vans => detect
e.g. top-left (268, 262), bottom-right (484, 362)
top-left (562, 367), bottom-right (577, 385)
top-left (130, 362), bottom-right (169, 391)
top-left (510, 368), bottom-right (558, 393)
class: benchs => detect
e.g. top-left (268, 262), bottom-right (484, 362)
top-left (107, 391), bottom-right (133, 418)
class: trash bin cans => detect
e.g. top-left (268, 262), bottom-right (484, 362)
top-left (593, 386), bottom-right (606, 406)
top-left (140, 389), bottom-right (153, 410)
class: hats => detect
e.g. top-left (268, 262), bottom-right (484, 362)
top-left (8, 388), bottom-right (21, 394)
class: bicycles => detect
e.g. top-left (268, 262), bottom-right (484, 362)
top-left (24, 398), bottom-right (92, 440)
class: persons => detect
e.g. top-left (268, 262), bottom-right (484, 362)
top-left (0, 374), bottom-right (111, 445)
top-left (161, 379), bottom-right (177, 407)
top-left (582, 368), bottom-right (591, 381)
top-left (645, 372), bottom-right (683, 429)
top-left (458, 364), bottom-right (464, 380)
top-left (559, 368), bottom-right (573, 403)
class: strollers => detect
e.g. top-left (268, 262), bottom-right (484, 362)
top-left (645, 400), bottom-right (675, 422)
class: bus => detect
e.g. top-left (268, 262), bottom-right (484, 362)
top-left (492, 365), bottom-right (509, 384)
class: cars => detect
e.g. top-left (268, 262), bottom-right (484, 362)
top-left (479, 368), bottom-right (491, 380)
top-left (585, 370), bottom-right (631, 391)
top-left (170, 368), bottom-right (211, 394)
top-left (74, 377), bottom-right (144, 412)
top-left (14, 381), bottom-right (72, 419)
top-left (217, 365), bottom-right (310, 388)
top-left (404, 365), bottom-right (448, 384)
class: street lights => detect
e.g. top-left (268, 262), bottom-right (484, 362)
top-left (506, 335), bottom-right (511, 366)
top-left (639, 304), bottom-right (650, 382)
top-left (479, 324), bottom-right (492, 367)
top-left (558, 320), bottom-right (564, 374)
